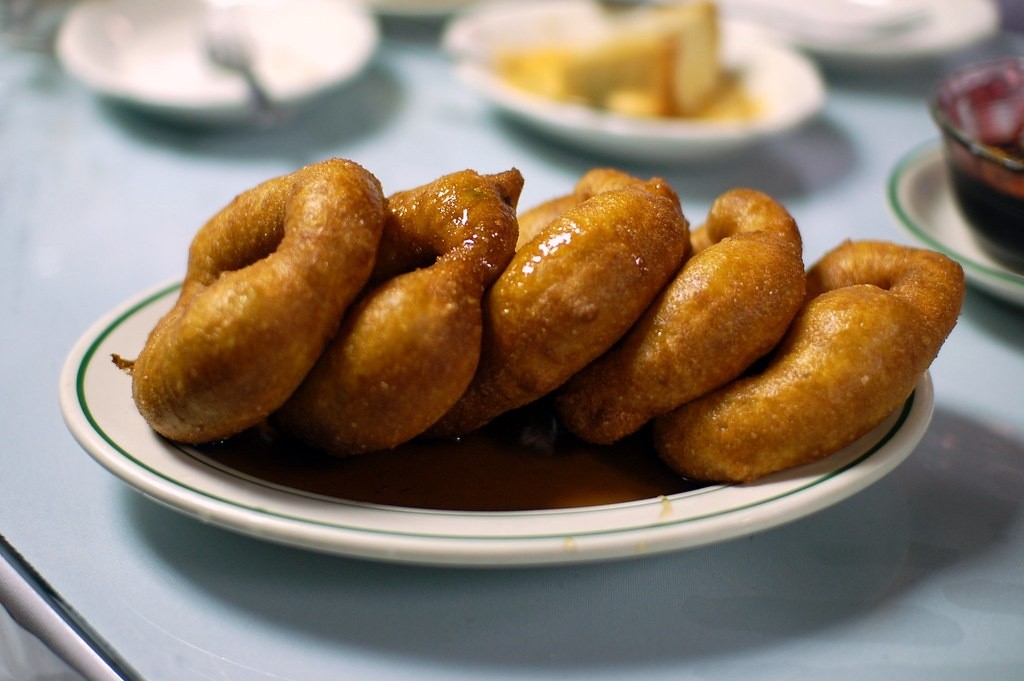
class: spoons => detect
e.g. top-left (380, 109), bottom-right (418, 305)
top-left (204, 32), bottom-right (280, 115)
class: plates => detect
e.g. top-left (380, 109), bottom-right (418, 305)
top-left (444, 0), bottom-right (826, 161)
top-left (739, 0), bottom-right (1001, 73)
top-left (57, 270), bottom-right (934, 566)
top-left (885, 135), bottom-right (1024, 304)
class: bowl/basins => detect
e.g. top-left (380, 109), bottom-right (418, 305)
top-left (54, 0), bottom-right (379, 128)
top-left (926, 57), bottom-right (1024, 275)
